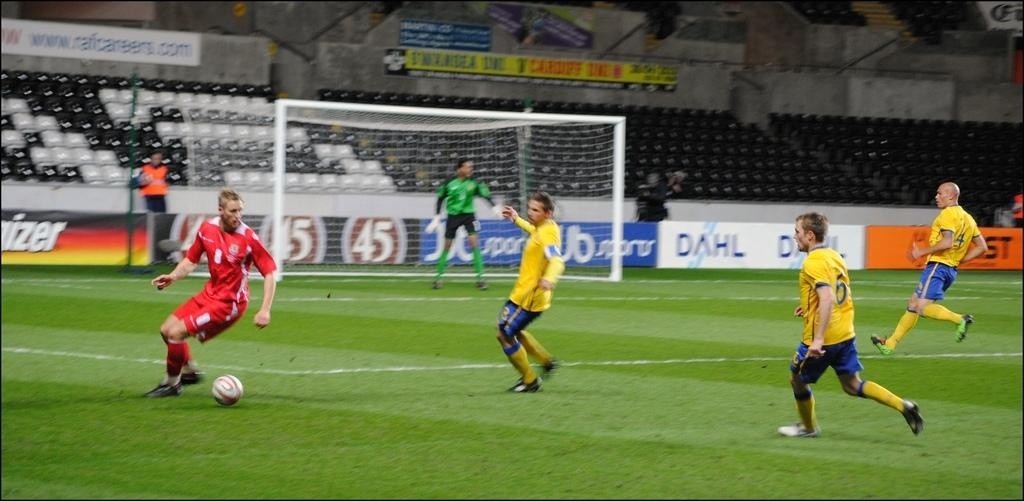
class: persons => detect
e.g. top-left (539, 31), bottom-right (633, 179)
top-left (495, 189), bottom-right (567, 394)
top-left (777, 211), bottom-right (927, 439)
top-left (872, 180), bottom-right (989, 356)
top-left (429, 157), bottom-right (498, 290)
top-left (142, 189), bottom-right (278, 401)
top-left (1011, 192), bottom-right (1024, 228)
top-left (637, 171), bottom-right (667, 222)
top-left (129, 150), bottom-right (175, 212)
top-left (667, 169), bottom-right (697, 199)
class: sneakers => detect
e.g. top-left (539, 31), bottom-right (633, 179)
top-left (181, 368), bottom-right (203, 384)
top-left (544, 356), bottom-right (558, 373)
top-left (145, 383), bottom-right (182, 397)
top-left (509, 377), bottom-right (542, 391)
top-left (871, 334), bottom-right (894, 355)
top-left (903, 400), bottom-right (925, 434)
top-left (955, 315), bottom-right (974, 342)
top-left (778, 424), bottom-right (818, 436)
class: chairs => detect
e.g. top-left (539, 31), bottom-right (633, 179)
top-left (1, 68), bottom-right (1023, 211)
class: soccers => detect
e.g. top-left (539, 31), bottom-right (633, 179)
top-left (213, 373), bottom-right (243, 407)
